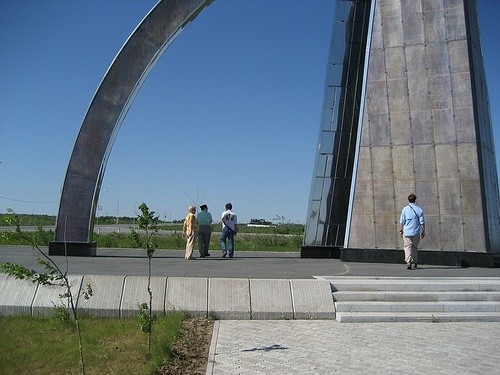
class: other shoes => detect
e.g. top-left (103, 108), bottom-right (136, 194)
top-left (205, 254), bottom-right (210, 256)
top-left (228, 252), bottom-right (233, 258)
top-left (185, 256), bottom-right (196, 260)
top-left (221, 252), bottom-right (227, 257)
top-left (199, 255), bottom-right (205, 258)
top-left (411, 263), bottom-right (416, 269)
top-left (407, 262), bottom-right (411, 269)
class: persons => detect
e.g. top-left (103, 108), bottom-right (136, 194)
top-left (220, 203), bottom-right (238, 258)
top-left (182, 205), bottom-right (199, 260)
top-left (196, 203), bottom-right (213, 257)
top-left (399, 194), bottom-right (426, 270)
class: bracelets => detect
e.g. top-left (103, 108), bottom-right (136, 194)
top-left (400, 230), bottom-right (402, 232)
